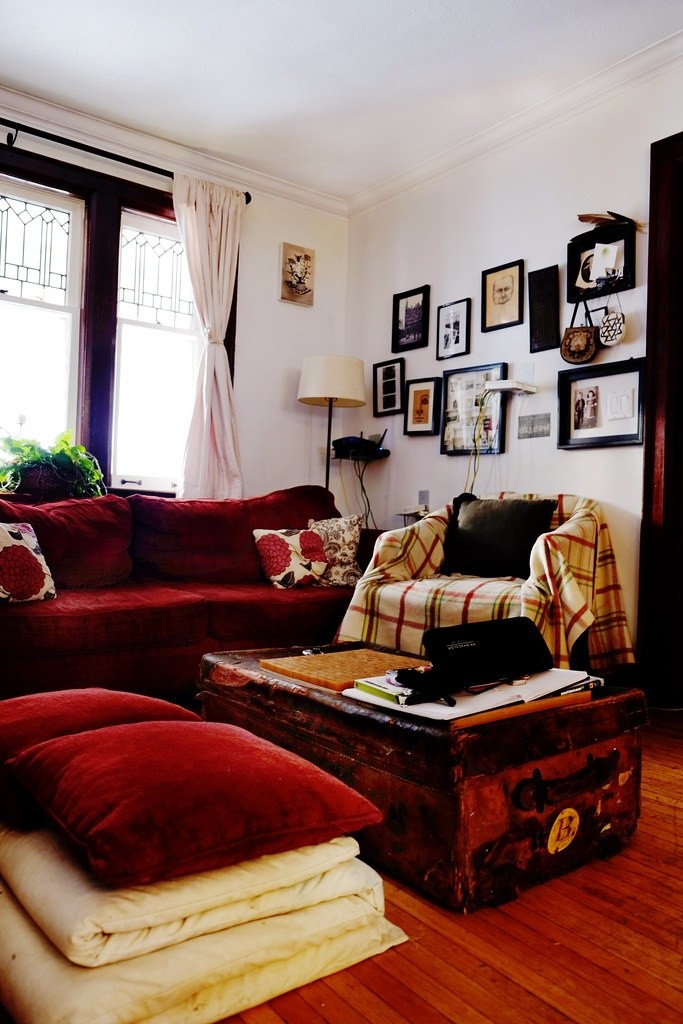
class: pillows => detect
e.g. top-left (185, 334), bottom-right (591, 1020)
top-left (438, 492), bottom-right (558, 579)
top-left (4, 721), bottom-right (385, 888)
top-left (0, 688), bottom-right (202, 825)
top-left (253, 529), bottom-right (331, 588)
top-left (0, 523), bottom-right (57, 605)
top-left (308, 512), bottom-right (364, 586)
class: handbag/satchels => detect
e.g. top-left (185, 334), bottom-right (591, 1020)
top-left (599, 310), bottom-right (626, 347)
top-left (560, 324), bottom-right (599, 365)
top-left (422, 616), bottom-right (555, 689)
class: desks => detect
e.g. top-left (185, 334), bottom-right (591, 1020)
top-left (194, 641), bottom-right (645, 914)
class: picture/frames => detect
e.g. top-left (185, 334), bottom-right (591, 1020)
top-left (436, 297), bottom-right (471, 360)
top-left (439, 363), bottom-right (508, 456)
top-left (373, 357), bottom-right (404, 417)
top-left (481, 259), bottom-right (523, 332)
top-left (557, 357), bottom-right (644, 450)
top-left (391, 284), bottom-right (430, 352)
top-left (566, 223), bottom-right (636, 305)
top-left (403, 376), bottom-right (440, 435)
top-left (278, 241), bottom-right (316, 307)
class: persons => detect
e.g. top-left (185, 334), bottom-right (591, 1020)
top-left (575, 390), bottom-right (598, 429)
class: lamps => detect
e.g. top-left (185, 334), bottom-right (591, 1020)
top-left (298, 355), bottom-right (367, 489)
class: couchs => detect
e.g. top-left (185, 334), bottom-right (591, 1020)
top-left (336, 493), bottom-right (635, 673)
top-left (0, 486), bottom-right (392, 704)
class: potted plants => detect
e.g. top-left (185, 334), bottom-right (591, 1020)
top-left (0, 432), bottom-right (108, 501)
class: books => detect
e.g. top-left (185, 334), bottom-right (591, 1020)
top-left (341, 668), bottom-right (604, 720)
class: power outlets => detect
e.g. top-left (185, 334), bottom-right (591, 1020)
top-left (369, 434), bottom-right (381, 443)
